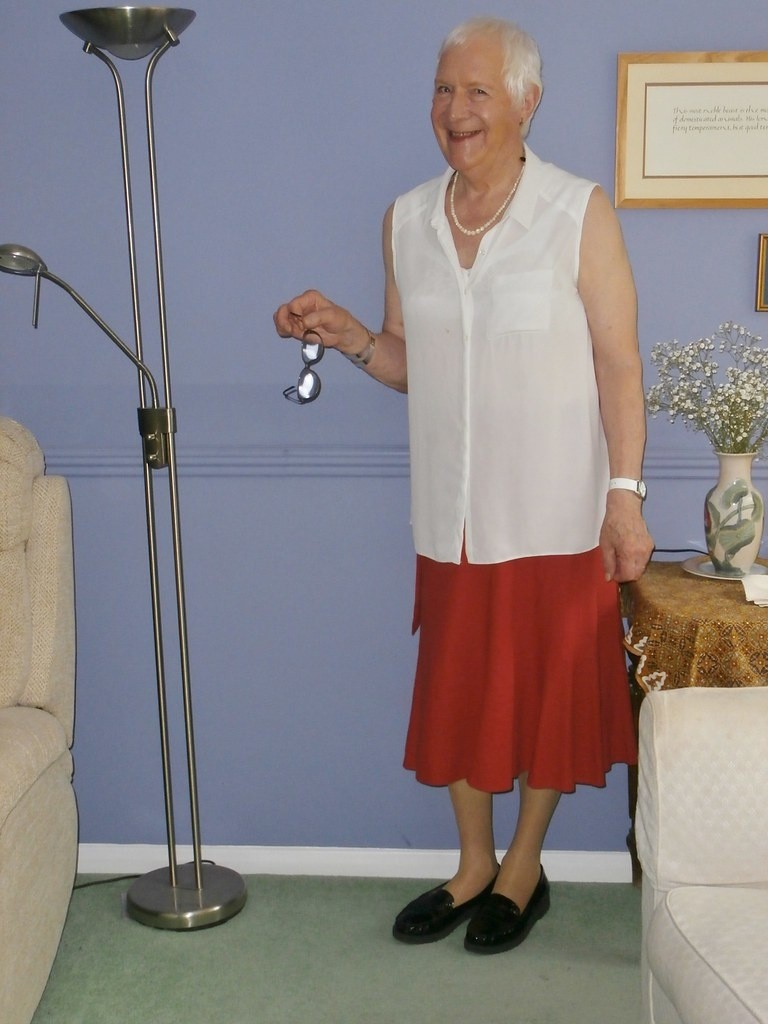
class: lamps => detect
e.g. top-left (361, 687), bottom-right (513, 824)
top-left (0, 4), bottom-right (249, 932)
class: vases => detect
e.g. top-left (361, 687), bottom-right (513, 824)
top-left (705, 452), bottom-right (763, 577)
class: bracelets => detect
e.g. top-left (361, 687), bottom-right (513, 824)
top-left (341, 328), bottom-right (375, 369)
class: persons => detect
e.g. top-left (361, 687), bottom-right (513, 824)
top-left (272, 18), bottom-right (656, 953)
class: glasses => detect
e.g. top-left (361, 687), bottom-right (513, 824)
top-left (282, 311), bottom-right (325, 404)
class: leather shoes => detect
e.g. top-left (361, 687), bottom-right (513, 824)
top-left (393, 862), bottom-right (500, 945)
top-left (463, 863), bottom-right (550, 954)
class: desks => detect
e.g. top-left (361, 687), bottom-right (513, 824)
top-left (618, 561), bottom-right (768, 893)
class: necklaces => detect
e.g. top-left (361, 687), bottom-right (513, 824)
top-left (450, 165), bottom-right (525, 236)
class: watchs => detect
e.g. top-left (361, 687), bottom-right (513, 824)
top-left (609, 478), bottom-right (648, 501)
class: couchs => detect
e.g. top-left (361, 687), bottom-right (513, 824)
top-left (637, 686), bottom-right (768, 1024)
top-left (0, 417), bottom-right (75, 1024)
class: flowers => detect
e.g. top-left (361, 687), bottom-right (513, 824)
top-left (645, 322), bottom-right (768, 453)
